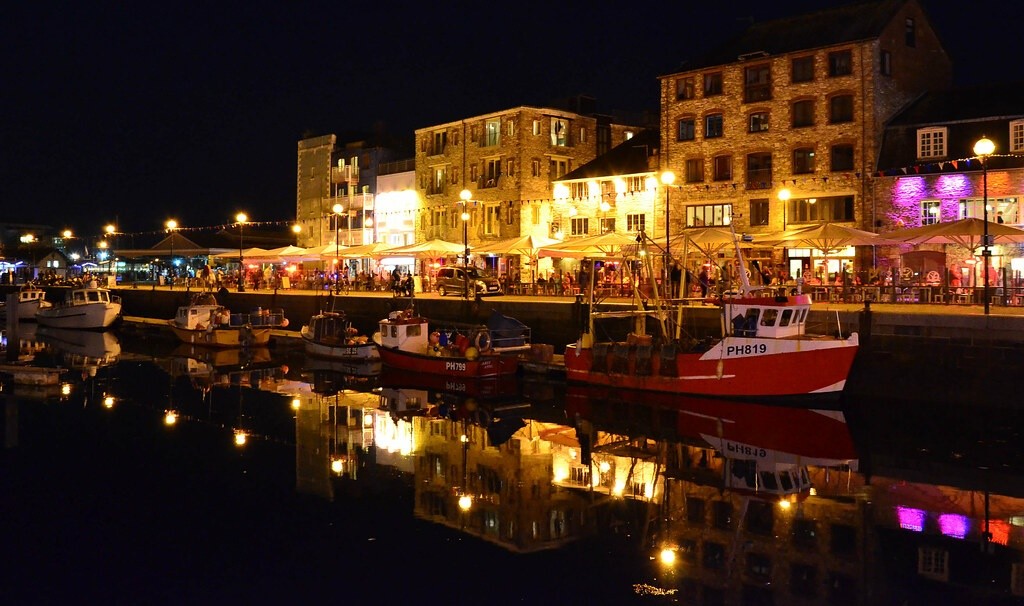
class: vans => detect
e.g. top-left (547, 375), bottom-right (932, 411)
top-left (436, 266), bottom-right (500, 297)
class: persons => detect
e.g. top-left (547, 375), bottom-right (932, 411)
top-left (148, 264), bottom-right (201, 292)
top-left (207, 305), bottom-right (231, 331)
top-left (336, 310), bottom-right (350, 345)
top-left (997, 212), bottom-right (1005, 224)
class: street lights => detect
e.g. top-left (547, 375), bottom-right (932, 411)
top-left (237, 213), bottom-right (246, 292)
top-left (660, 171), bottom-right (675, 298)
top-left (972, 135), bottom-right (996, 314)
top-left (167, 221), bottom-right (176, 290)
top-left (332, 203), bottom-right (344, 295)
top-left (779, 188), bottom-right (792, 231)
top-left (460, 189), bottom-right (472, 299)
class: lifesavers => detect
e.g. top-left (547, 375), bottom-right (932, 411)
top-left (475, 332), bottom-right (490, 352)
top-left (210, 307), bottom-right (229, 329)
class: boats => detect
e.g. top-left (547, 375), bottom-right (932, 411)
top-left (372, 310), bottom-right (532, 378)
top-left (301, 310), bottom-right (372, 360)
top-left (0, 280), bottom-right (52, 320)
top-left (564, 228), bottom-right (860, 395)
top-left (167, 294), bottom-right (290, 347)
top-left (34, 280), bottom-right (121, 329)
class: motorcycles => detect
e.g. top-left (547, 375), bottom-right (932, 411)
top-left (31, 275), bottom-right (105, 287)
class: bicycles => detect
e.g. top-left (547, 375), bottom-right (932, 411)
top-left (333, 284), bottom-right (350, 296)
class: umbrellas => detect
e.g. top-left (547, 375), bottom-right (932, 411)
top-left (201, 217), bottom-right (1024, 309)
